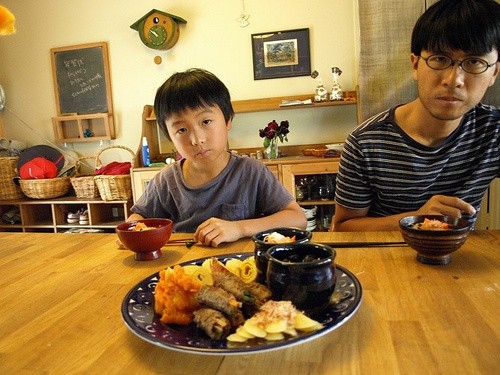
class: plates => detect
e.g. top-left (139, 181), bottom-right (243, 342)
top-left (120, 251), bottom-right (363, 356)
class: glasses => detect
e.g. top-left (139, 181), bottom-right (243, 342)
top-left (418, 54), bottom-right (498, 75)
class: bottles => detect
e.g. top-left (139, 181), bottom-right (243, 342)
top-left (322, 207), bottom-right (332, 229)
top-left (142, 137), bottom-right (151, 167)
top-left (295, 184), bottom-right (304, 202)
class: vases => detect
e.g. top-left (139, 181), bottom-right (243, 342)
top-left (266, 137), bottom-right (279, 159)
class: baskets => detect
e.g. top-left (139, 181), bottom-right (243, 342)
top-left (0, 156), bottom-right (20, 200)
top-left (17, 177), bottom-right (70, 199)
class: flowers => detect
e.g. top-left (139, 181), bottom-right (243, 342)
top-left (259, 120), bottom-right (290, 146)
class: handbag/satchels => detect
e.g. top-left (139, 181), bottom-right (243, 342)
top-left (70, 156), bottom-right (103, 198)
top-left (93, 146), bottom-right (145, 200)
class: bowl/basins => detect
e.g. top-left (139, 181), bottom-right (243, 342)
top-left (266, 242), bottom-right (336, 316)
top-left (302, 148), bottom-right (315, 156)
top-left (311, 149), bottom-right (327, 156)
top-left (116, 218), bottom-right (173, 261)
top-left (399, 214), bottom-right (472, 265)
top-left (325, 143), bottom-right (345, 152)
top-left (252, 228), bottom-right (312, 281)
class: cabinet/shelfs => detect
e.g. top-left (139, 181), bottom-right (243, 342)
top-left (130, 84), bottom-right (362, 232)
top-left (0, 199), bottom-right (131, 234)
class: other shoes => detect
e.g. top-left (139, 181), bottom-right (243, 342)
top-left (67, 210), bottom-right (80, 218)
top-left (79, 210), bottom-right (89, 220)
top-left (67, 218), bottom-right (79, 223)
top-left (79, 220), bottom-right (89, 224)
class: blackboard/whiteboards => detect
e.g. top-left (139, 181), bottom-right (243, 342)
top-left (50, 42), bottom-right (113, 118)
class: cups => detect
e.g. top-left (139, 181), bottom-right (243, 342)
top-left (317, 186), bottom-right (330, 201)
top-left (232, 150), bottom-right (268, 160)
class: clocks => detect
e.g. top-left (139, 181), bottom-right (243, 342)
top-left (130, 8), bottom-right (187, 51)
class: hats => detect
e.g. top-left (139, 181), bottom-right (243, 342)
top-left (18, 144), bottom-right (64, 179)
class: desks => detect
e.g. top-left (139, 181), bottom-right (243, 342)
top-left (0, 232), bottom-right (500, 375)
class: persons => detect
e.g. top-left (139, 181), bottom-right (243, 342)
top-left (117, 68), bottom-right (307, 247)
top-left (334, 0), bottom-right (500, 231)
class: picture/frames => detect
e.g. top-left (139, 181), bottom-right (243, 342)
top-left (251, 27), bottom-right (312, 81)
